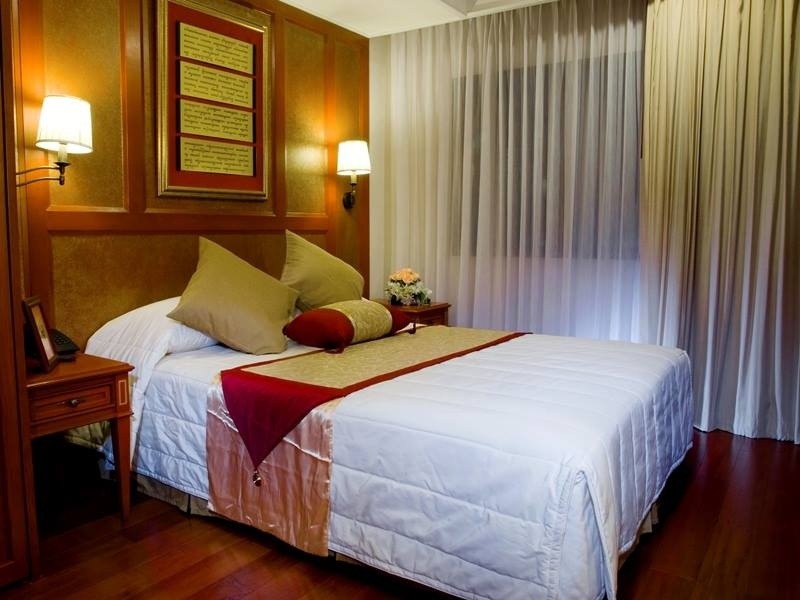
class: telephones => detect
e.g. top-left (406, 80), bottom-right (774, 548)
top-left (23, 322), bottom-right (81, 360)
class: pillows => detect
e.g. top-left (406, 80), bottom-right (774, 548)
top-left (282, 299), bottom-right (411, 349)
top-left (166, 236), bottom-right (299, 354)
top-left (280, 229), bottom-right (364, 313)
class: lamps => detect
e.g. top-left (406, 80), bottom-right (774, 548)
top-left (336, 139), bottom-right (371, 209)
top-left (16, 95), bottom-right (93, 186)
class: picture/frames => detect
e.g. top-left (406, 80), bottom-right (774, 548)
top-left (22, 295), bottom-right (59, 372)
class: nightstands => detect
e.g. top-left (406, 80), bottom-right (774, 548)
top-left (372, 298), bottom-right (452, 326)
top-left (27, 354), bottom-right (136, 520)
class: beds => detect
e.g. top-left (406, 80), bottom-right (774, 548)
top-left (65, 295), bottom-right (693, 600)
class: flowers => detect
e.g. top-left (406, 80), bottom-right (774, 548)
top-left (388, 267), bottom-right (432, 307)
